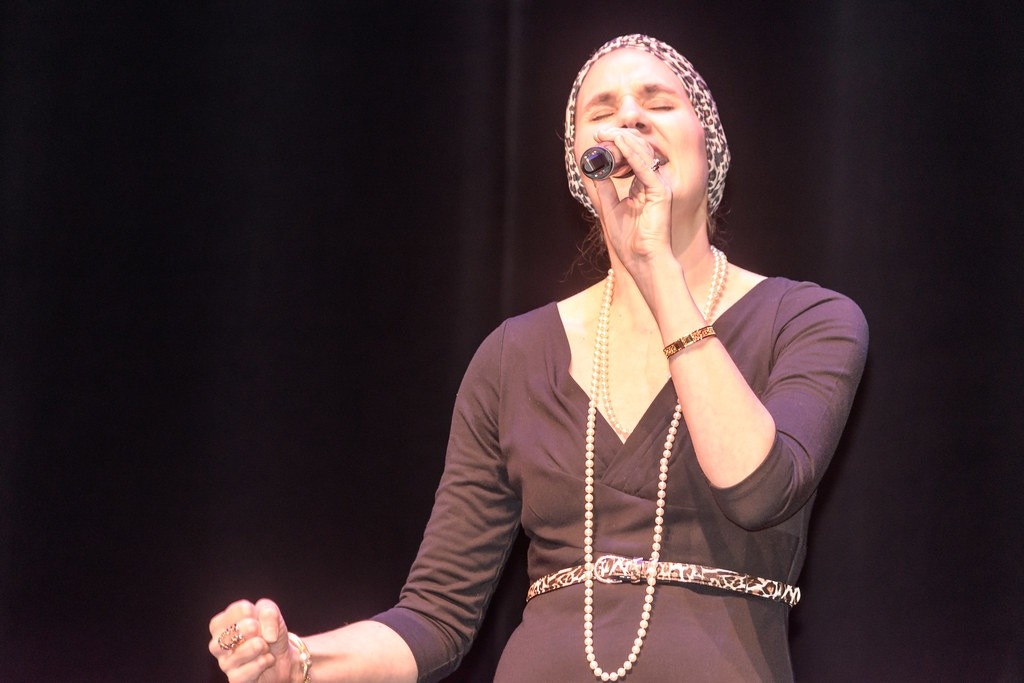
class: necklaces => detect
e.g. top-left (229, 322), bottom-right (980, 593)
top-left (581, 246), bottom-right (729, 681)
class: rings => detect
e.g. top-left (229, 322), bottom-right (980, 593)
top-left (217, 624), bottom-right (246, 652)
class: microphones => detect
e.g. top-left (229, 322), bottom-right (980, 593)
top-left (580, 139), bottom-right (635, 179)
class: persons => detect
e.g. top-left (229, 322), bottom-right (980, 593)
top-left (208, 32), bottom-right (870, 683)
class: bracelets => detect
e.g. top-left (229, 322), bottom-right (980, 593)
top-left (663, 324), bottom-right (717, 359)
top-left (288, 631), bottom-right (314, 683)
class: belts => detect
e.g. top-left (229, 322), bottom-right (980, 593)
top-left (525, 554), bottom-right (801, 607)
